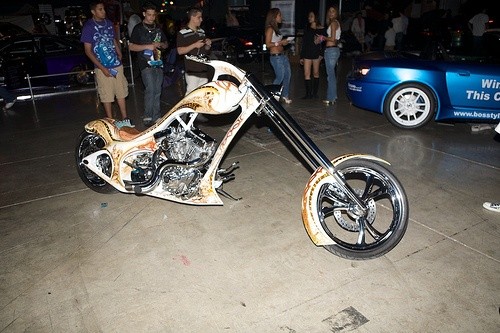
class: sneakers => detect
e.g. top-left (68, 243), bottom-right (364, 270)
top-left (121, 116), bottom-right (135, 127)
top-left (482, 201), bottom-right (500, 213)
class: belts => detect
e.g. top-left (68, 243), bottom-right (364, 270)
top-left (269, 51), bottom-right (287, 56)
top-left (324, 45), bottom-right (339, 48)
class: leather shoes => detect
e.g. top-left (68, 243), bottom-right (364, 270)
top-left (143, 118), bottom-right (156, 127)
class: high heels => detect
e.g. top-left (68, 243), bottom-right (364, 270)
top-left (281, 96), bottom-right (293, 104)
top-left (322, 97), bottom-right (338, 105)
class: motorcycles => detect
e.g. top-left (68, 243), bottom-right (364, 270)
top-left (75, 54), bottom-right (410, 261)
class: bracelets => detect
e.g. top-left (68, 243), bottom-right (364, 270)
top-left (275, 42), bottom-right (280, 46)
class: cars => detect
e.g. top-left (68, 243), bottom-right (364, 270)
top-left (344, 29), bottom-right (500, 130)
top-left (0, 31), bottom-right (95, 92)
top-left (164, 26), bottom-right (296, 63)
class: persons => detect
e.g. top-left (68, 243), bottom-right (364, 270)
top-left (81, 1), bottom-right (134, 124)
top-left (0, 85), bottom-right (17, 109)
top-left (264, 8), bottom-right (293, 105)
top-left (129, 6), bottom-right (168, 127)
top-left (483, 122), bottom-right (500, 213)
top-left (314, 7), bottom-right (341, 104)
top-left (42, 12), bottom-right (59, 36)
top-left (384, 23), bottom-right (395, 51)
top-left (176, 8), bottom-right (212, 128)
top-left (299, 11), bottom-right (326, 100)
top-left (468, 8), bottom-right (489, 63)
top-left (351, 12), bottom-right (365, 52)
top-left (342, 9), bottom-right (410, 49)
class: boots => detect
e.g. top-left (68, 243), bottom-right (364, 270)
top-left (301, 77), bottom-right (321, 99)
